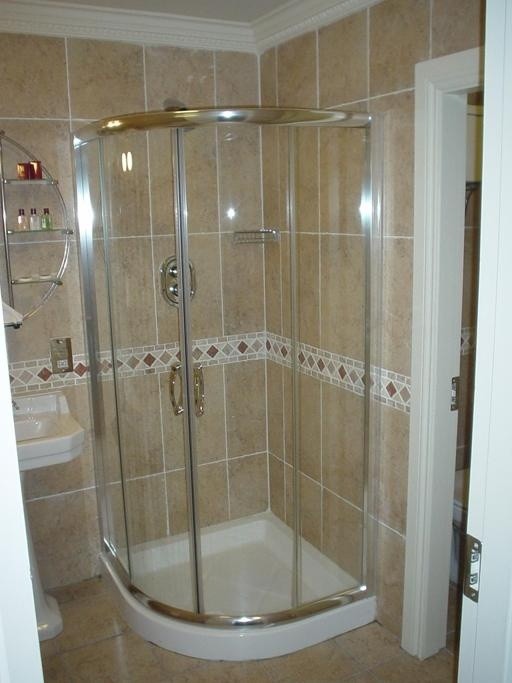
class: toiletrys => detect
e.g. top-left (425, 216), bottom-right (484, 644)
top-left (17, 208), bottom-right (29, 229)
top-left (28, 207), bottom-right (41, 228)
top-left (41, 207), bottom-right (53, 228)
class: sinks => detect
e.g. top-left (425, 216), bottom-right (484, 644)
top-left (13, 391), bottom-right (83, 472)
